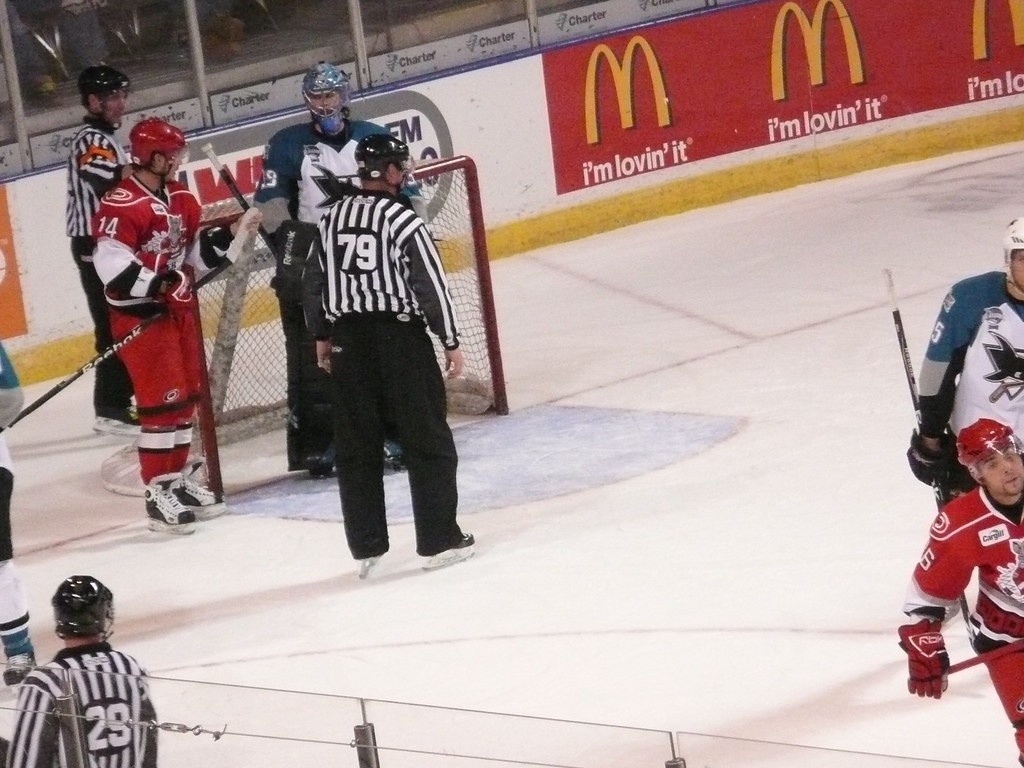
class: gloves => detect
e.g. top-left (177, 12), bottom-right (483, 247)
top-left (898, 618), bottom-right (949, 700)
top-left (906, 429), bottom-right (951, 488)
top-left (155, 271), bottom-right (198, 319)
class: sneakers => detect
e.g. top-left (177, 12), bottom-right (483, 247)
top-left (171, 462), bottom-right (228, 519)
top-left (3, 651), bottom-right (35, 695)
top-left (359, 551), bottom-right (383, 579)
top-left (942, 599), bottom-right (962, 628)
top-left (143, 477), bottom-right (197, 533)
top-left (382, 441), bottom-right (408, 475)
top-left (305, 454), bottom-right (339, 478)
top-left (422, 534), bottom-right (476, 568)
top-left (93, 403), bottom-right (139, 436)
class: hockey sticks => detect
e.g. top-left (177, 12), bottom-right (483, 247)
top-left (947, 638), bottom-right (1023, 673)
top-left (200, 141), bottom-right (282, 259)
top-left (7, 206), bottom-right (260, 430)
top-left (880, 266), bottom-right (977, 656)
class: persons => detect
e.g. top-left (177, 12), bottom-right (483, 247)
top-left (6, 0), bottom-right (106, 89)
top-left (65, 65), bottom-right (142, 435)
top-left (298, 135), bottom-right (477, 580)
top-left (92, 119), bottom-right (262, 534)
top-left (908, 217), bottom-right (1024, 507)
top-left (0, 576), bottom-right (158, 768)
top-left (899, 419), bottom-right (1023, 765)
top-left (0, 342), bottom-right (38, 691)
top-left (253, 61), bottom-right (426, 480)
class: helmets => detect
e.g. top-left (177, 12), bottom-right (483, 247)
top-left (955, 418), bottom-right (1015, 465)
top-left (301, 62), bottom-right (350, 133)
top-left (50, 574), bottom-right (113, 638)
top-left (130, 119), bottom-right (186, 167)
top-left (354, 134), bottom-right (410, 182)
top-left (1002, 217), bottom-right (1024, 262)
top-left (77, 65), bottom-right (129, 97)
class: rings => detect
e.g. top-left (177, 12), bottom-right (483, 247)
top-left (317, 363), bottom-right (322, 368)
top-left (323, 360), bottom-right (330, 363)
